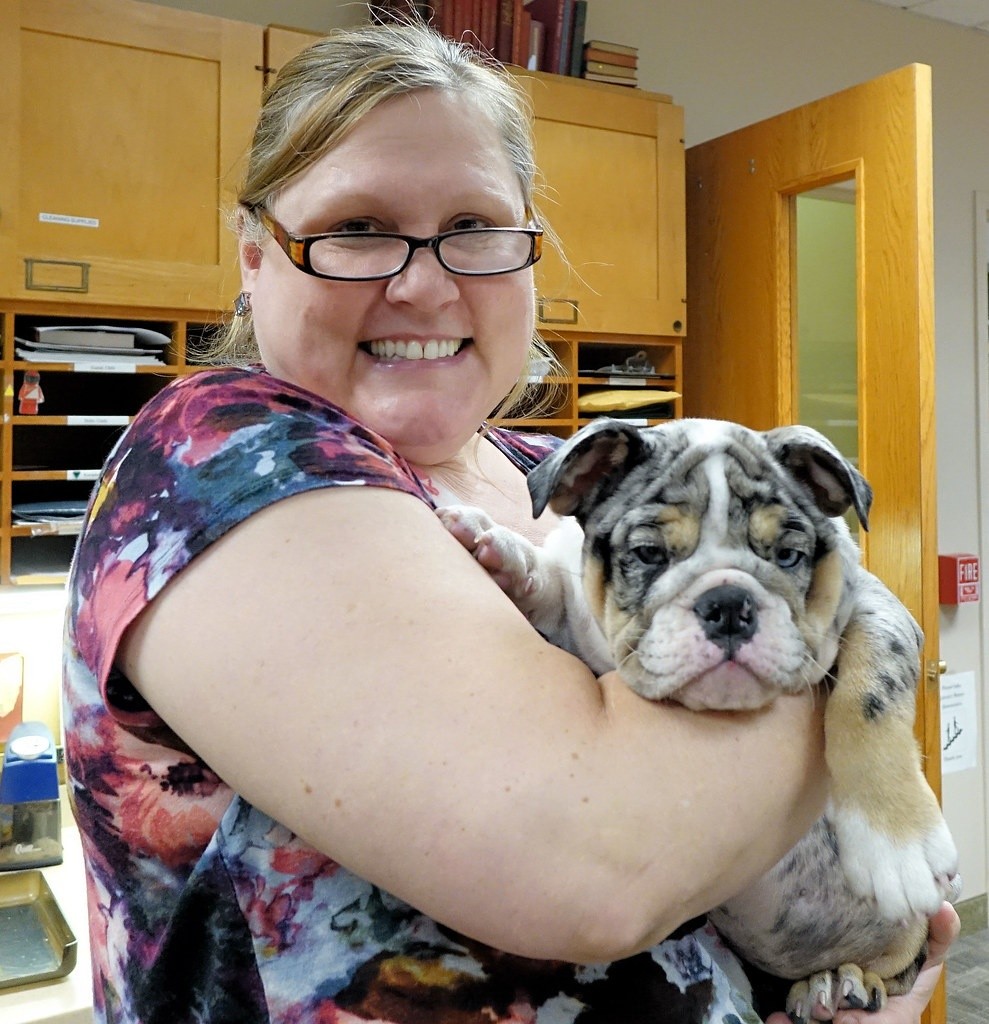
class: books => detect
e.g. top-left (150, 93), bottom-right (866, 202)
top-left (370, 0), bottom-right (639, 89)
top-left (36, 328), bottom-right (138, 348)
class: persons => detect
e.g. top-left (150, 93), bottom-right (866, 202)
top-left (60, 21), bottom-right (964, 1023)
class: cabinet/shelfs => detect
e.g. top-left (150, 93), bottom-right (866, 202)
top-left (1, 0), bottom-right (350, 312)
top-left (1, 298), bottom-right (267, 588)
top-left (486, 329), bottom-right (684, 442)
top-left (510, 70), bottom-right (688, 342)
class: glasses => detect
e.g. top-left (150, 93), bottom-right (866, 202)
top-left (251, 199), bottom-right (545, 282)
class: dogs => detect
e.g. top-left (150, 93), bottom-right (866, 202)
top-left (432, 417), bottom-right (964, 1023)
